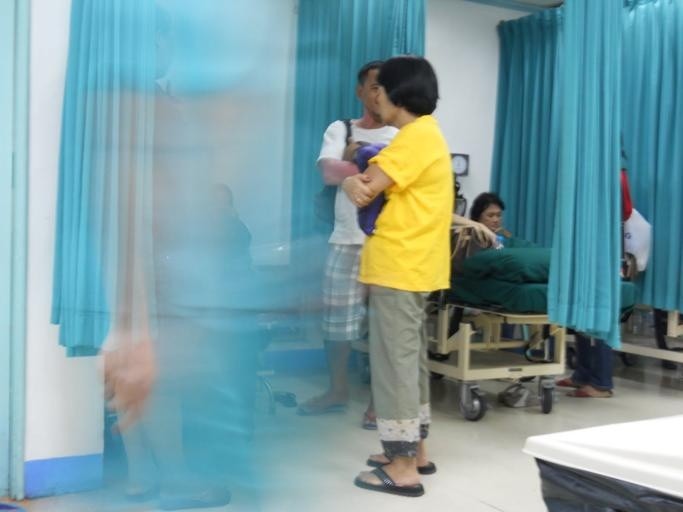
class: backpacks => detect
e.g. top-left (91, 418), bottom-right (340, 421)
top-left (350, 140), bottom-right (393, 236)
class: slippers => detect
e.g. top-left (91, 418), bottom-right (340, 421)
top-left (362, 409), bottom-right (379, 430)
top-left (354, 464), bottom-right (424, 497)
top-left (366, 451), bottom-right (437, 475)
top-left (496, 382), bottom-right (543, 408)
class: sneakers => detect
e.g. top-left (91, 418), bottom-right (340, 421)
top-left (556, 378), bottom-right (613, 398)
top-left (296, 398), bottom-right (348, 415)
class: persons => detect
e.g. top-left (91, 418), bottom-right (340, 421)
top-left (297, 63), bottom-right (404, 432)
top-left (556, 131), bottom-right (633, 398)
top-left (97, 1), bottom-right (270, 511)
top-left (467, 193), bottom-right (514, 253)
top-left (340, 61), bottom-right (451, 497)
